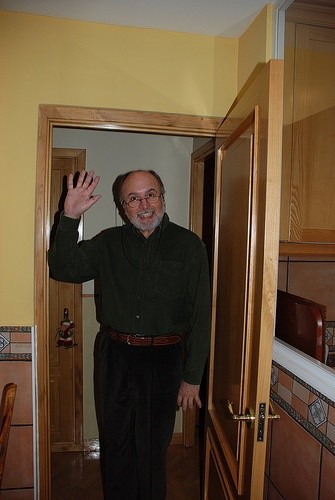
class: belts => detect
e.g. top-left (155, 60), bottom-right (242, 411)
top-left (98, 326), bottom-right (181, 346)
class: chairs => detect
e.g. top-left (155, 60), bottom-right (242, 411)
top-left (0, 383), bottom-right (17, 487)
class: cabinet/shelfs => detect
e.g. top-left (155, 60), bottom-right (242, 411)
top-left (279, 3), bottom-right (335, 260)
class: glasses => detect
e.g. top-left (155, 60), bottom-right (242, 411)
top-left (123, 192), bottom-right (163, 208)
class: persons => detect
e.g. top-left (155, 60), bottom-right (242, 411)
top-left (47, 167), bottom-right (213, 500)
